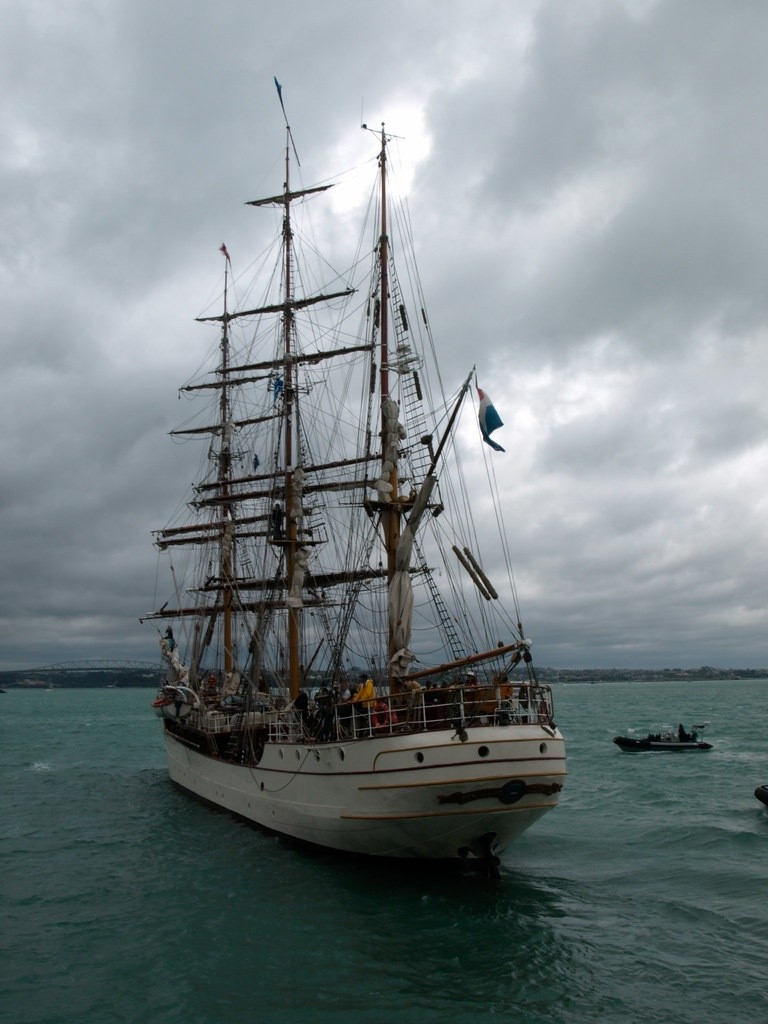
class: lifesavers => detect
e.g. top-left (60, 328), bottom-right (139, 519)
top-left (369, 704), bottom-right (394, 731)
top-left (152, 698), bottom-right (173, 707)
top-left (537, 698), bottom-right (552, 720)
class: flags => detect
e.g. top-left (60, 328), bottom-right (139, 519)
top-left (475, 372), bottom-right (507, 453)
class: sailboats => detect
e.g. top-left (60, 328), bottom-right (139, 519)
top-left (134, 72), bottom-right (570, 879)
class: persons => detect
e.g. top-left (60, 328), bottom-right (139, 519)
top-left (495, 673), bottom-right (514, 710)
top-left (300, 664), bottom-right (306, 683)
top-left (647, 733), bottom-right (662, 742)
top-left (258, 676), bottom-right (265, 692)
top-left (406, 669), bottom-right (481, 692)
top-left (208, 674), bottom-right (218, 688)
top-left (272, 503), bottom-right (283, 541)
top-left (165, 624), bottom-right (175, 652)
top-left (293, 673), bottom-right (377, 742)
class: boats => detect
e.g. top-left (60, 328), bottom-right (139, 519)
top-left (613, 725), bottom-right (715, 753)
